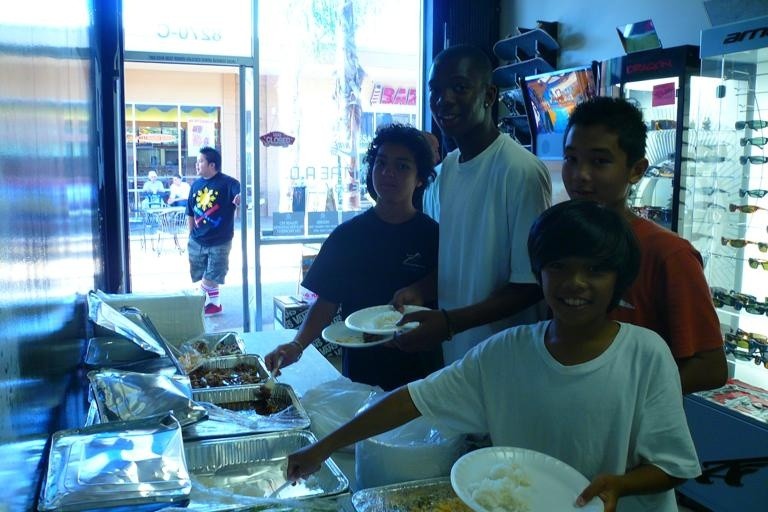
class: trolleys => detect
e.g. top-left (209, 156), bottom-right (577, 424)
top-left (147, 206), bottom-right (187, 256)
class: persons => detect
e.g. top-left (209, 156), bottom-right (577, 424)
top-left (391, 43), bottom-right (552, 368)
top-left (142, 171), bottom-right (166, 208)
top-left (265, 122), bottom-right (440, 388)
top-left (546, 93), bottom-right (728, 395)
top-left (167, 174), bottom-right (191, 225)
top-left (421, 131), bottom-right (441, 168)
top-left (185, 146), bottom-right (241, 317)
top-left (285, 199), bottom-right (703, 512)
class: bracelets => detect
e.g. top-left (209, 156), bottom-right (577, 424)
top-left (441, 307), bottom-right (453, 341)
top-left (290, 340), bottom-right (303, 359)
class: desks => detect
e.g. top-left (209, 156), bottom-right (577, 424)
top-left (210, 328), bottom-right (358, 511)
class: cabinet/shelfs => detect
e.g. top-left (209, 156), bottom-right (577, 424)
top-left (618, 46), bottom-right (757, 376)
top-left (489, 20), bottom-right (561, 158)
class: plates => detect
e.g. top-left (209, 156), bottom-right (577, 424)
top-left (321, 300), bottom-right (437, 352)
top-left (448, 444), bottom-right (607, 512)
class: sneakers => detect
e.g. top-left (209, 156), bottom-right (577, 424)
top-left (203, 301), bottom-right (223, 317)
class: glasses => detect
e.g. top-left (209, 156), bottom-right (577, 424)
top-left (649, 118), bottom-right (677, 130)
top-left (734, 119), bottom-right (768, 131)
top-left (710, 285), bottom-right (768, 317)
top-left (720, 237), bottom-right (753, 249)
top-left (738, 135), bottom-right (768, 149)
top-left (739, 189), bottom-right (768, 200)
top-left (739, 154), bottom-right (768, 166)
top-left (724, 326), bottom-right (768, 369)
top-left (747, 258), bottom-right (768, 272)
top-left (727, 203), bottom-right (767, 214)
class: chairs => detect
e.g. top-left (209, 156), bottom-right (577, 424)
top-left (128, 188), bottom-right (190, 257)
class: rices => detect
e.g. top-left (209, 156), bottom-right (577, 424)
top-left (472, 463), bottom-right (532, 512)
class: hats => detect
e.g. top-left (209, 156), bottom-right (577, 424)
top-left (148, 171), bottom-right (157, 179)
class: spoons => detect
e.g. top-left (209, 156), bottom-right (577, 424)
top-left (252, 351), bottom-right (286, 401)
